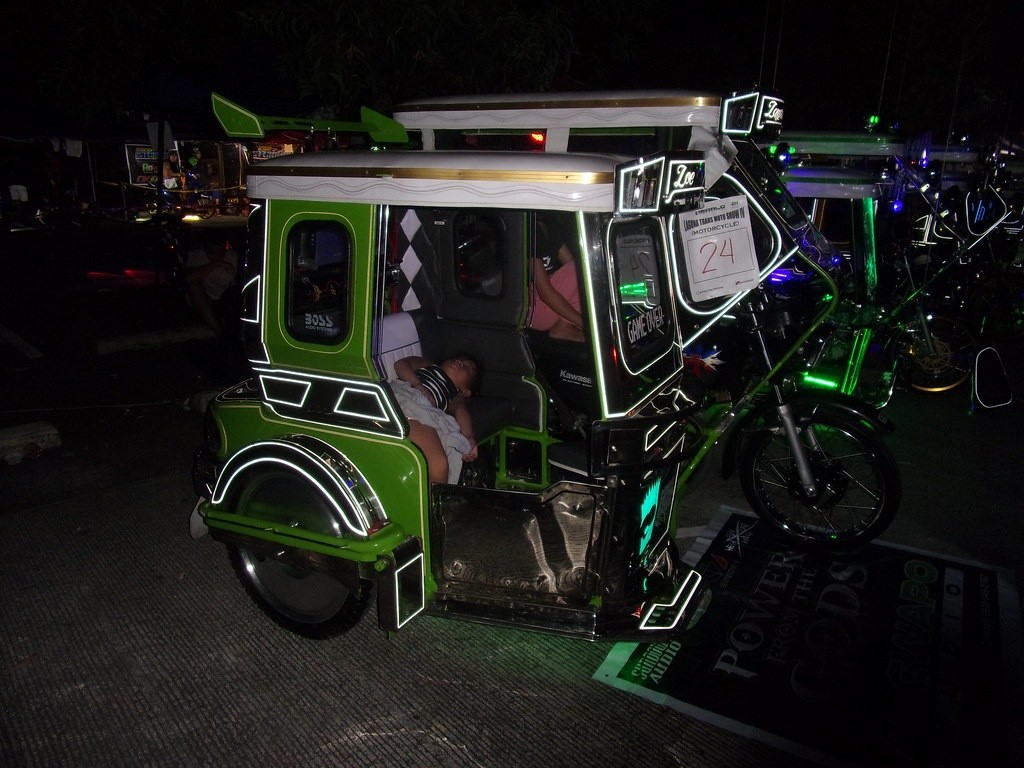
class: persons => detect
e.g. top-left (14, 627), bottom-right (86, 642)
top-left (388, 356), bottom-right (481, 486)
top-left (163, 147), bottom-right (223, 204)
top-left (462, 207), bottom-right (587, 344)
top-left (180, 249), bottom-right (326, 336)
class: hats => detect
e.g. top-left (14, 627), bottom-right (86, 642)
top-left (192, 147), bottom-right (199, 153)
top-left (170, 151), bottom-right (177, 156)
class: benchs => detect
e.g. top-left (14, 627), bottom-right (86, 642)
top-left (381, 309), bottom-right (518, 445)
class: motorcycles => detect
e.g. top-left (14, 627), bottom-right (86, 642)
top-left (144, 166), bottom-right (215, 219)
top-left (195, 84), bottom-right (1016, 644)
